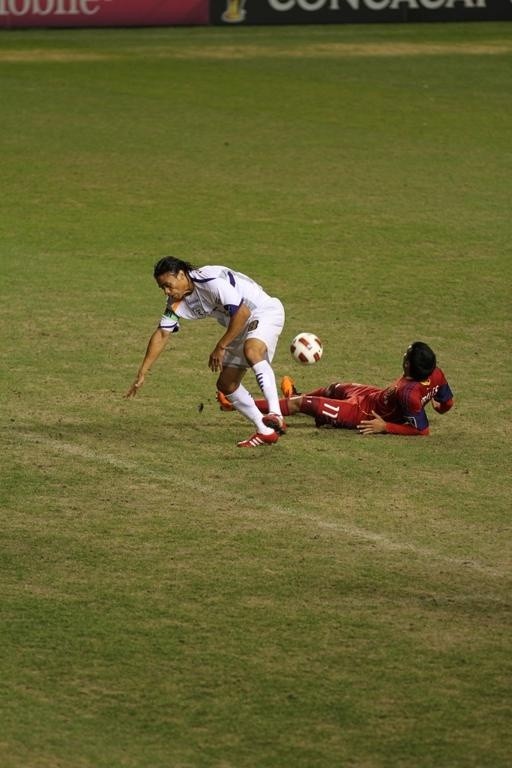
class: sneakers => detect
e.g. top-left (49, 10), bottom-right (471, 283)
top-left (263, 412), bottom-right (286, 436)
top-left (281, 376), bottom-right (296, 398)
top-left (238, 432), bottom-right (278, 449)
top-left (216, 389), bottom-right (234, 412)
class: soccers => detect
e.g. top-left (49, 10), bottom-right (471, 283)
top-left (291, 332), bottom-right (323, 365)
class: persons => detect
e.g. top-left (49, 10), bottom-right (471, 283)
top-left (215, 341), bottom-right (454, 435)
top-left (121, 255), bottom-right (287, 450)
